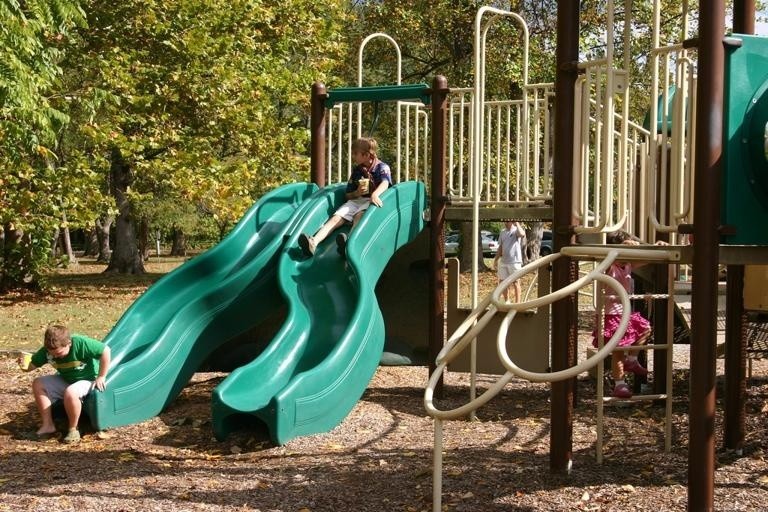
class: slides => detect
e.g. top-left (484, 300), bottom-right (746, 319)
top-left (52, 181), bottom-right (347, 431)
top-left (212, 180), bottom-right (428, 446)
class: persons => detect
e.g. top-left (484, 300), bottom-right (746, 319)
top-left (298, 138), bottom-right (393, 257)
top-left (494, 221), bottom-right (525, 304)
top-left (17, 325), bottom-right (112, 442)
top-left (591, 230), bottom-right (670, 398)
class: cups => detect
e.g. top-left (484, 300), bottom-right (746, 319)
top-left (19, 351), bottom-right (32, 370)
top-left (359, 179), bottom-right (369, 194)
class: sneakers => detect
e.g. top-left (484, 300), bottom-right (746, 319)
top-left (63, 428), bottom-right (80, 444)
top-left (297, 232), bottom-right (316, 257)
top-left (624, 357), bottom-right (649, 375)
top-left (335, 233), bottom-right (349, 259)
top-left (613, 384), bottom-right (633, 396)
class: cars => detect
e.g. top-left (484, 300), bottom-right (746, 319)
top-left (445, 230), bottom-right (553, 257)
